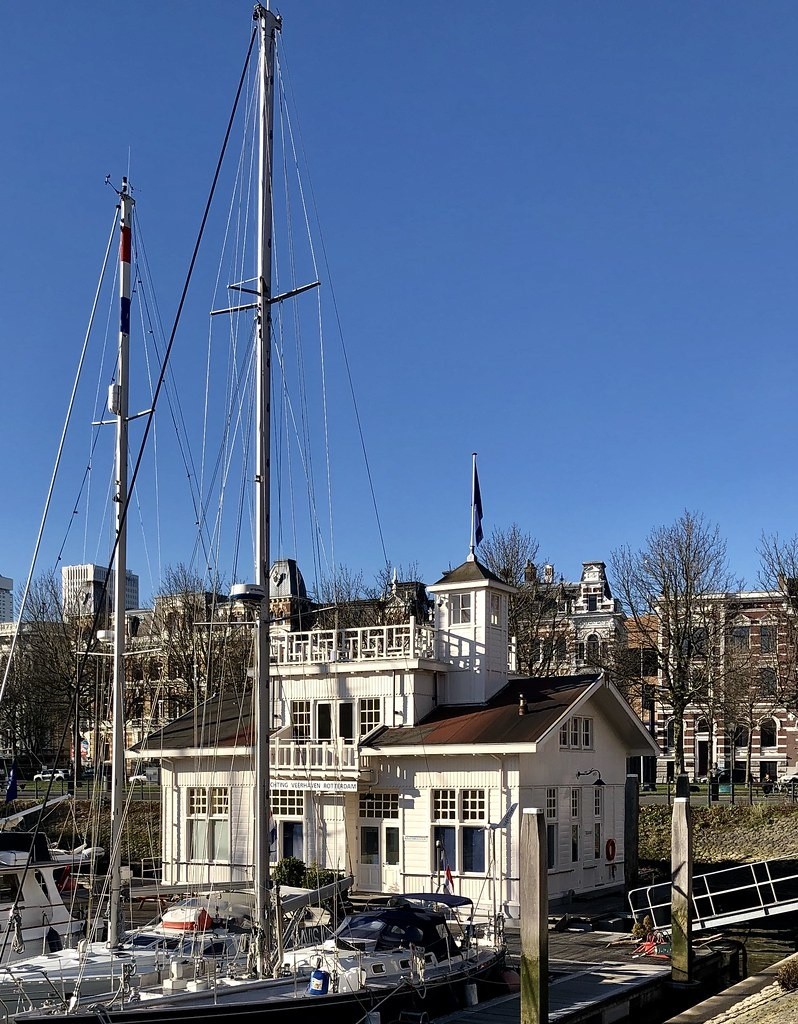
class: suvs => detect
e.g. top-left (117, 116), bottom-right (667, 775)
top-left (33, 768), bottom-right (69, 782)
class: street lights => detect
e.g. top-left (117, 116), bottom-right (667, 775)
top-left (638, 639), bottom-right (645, 788)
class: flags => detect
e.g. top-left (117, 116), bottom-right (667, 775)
top-left (445, 865), bottom-right (455, 892)
top-left (471, 461), bottom-right (484, 547)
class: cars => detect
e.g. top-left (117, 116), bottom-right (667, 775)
top-left (128, 772), bottom-right (147, 785)
top-left (778, 771), bottom-right (798, 787)
top-left (701, 766), bottom-right (753, 784)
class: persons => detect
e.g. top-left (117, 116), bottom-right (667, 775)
top-left (762, 773), bottom-right (773, 798)
top-left (710, 762), bottom-right (720, 779)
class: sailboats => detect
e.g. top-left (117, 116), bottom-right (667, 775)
top-left (0, 0), bottom-right (512, 1024)
